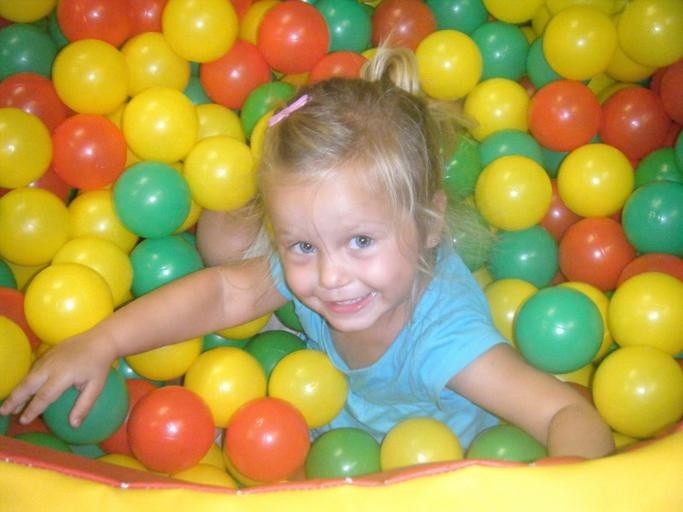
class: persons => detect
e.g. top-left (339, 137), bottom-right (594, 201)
top-left (0, 31), bottom-right (616, 459)
